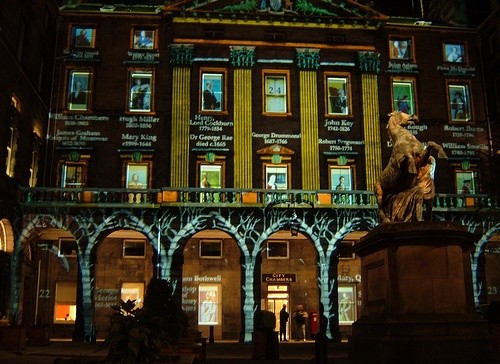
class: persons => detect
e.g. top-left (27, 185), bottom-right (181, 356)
top-left (294, 305), bottom-right (308, 341)
top-left (279, 305), bottom-right (289, 342)
top-left (309, 307), bottom-right (319, 339)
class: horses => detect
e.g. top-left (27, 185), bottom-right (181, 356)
top-left (373, 112), bottom-right (447, 224)
